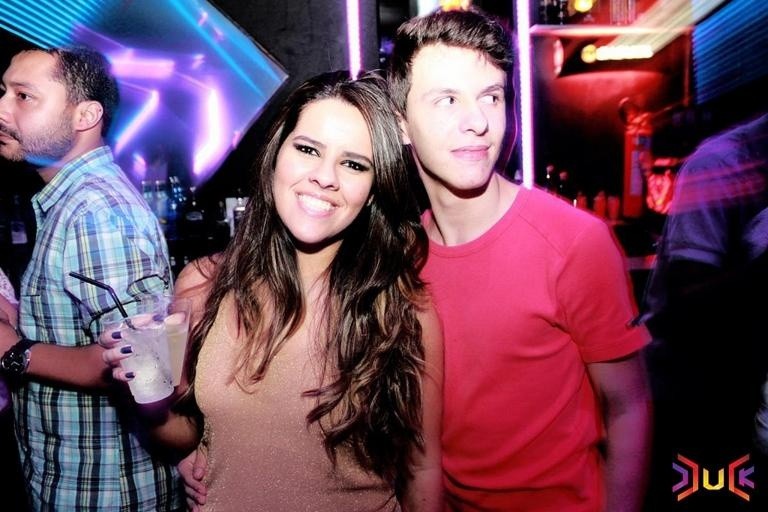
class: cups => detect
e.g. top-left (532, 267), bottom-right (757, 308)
top-left (100, 295), bottom-right (191, 406)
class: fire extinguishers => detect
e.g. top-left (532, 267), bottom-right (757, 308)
top-left (623, 111), bottom-right (653, 219)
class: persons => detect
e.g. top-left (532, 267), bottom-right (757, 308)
top-left (0, 39), bottom-right (184, 511)
top-left (174, 2), bottom-right (663, 510)
top-left (92, 68), bottom-right (448, 510)
top-left (649, 106), bottom-right (767, 511)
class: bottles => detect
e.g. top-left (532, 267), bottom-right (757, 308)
top-left (546, 164), bottom-right (571, 197)
top-left (142, 180), bottom-right (176, 232)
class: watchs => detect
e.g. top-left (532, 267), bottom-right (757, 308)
top-left (1, 337), bottom-right (40, 380)
